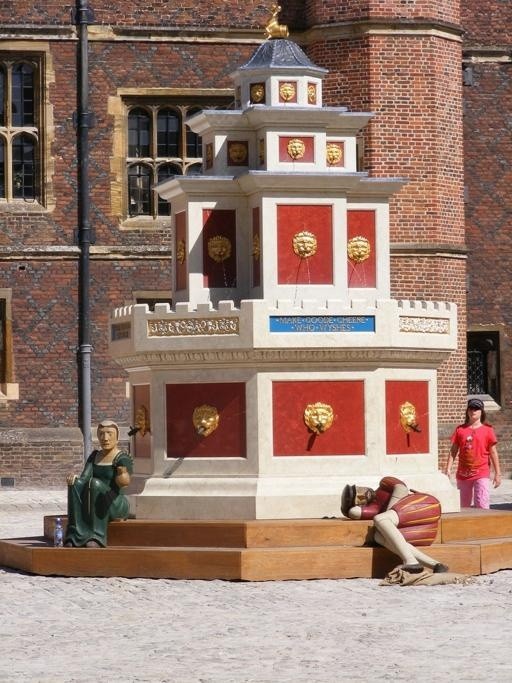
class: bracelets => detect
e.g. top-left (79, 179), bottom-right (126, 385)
top-left (496, 473), bottom-right (501, 477)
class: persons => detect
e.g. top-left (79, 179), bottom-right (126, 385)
top-left (343, 476), bottom-right (449, 573)
top-left (445, 398), bottom-right (502, 509)
top-left (65, 419), bottom-right (133, 547)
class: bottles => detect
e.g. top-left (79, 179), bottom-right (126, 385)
top-left (55, 516), bottom-right (64, 548)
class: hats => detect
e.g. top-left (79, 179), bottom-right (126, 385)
top-left (467, 399), bottom-right (484, 410)
